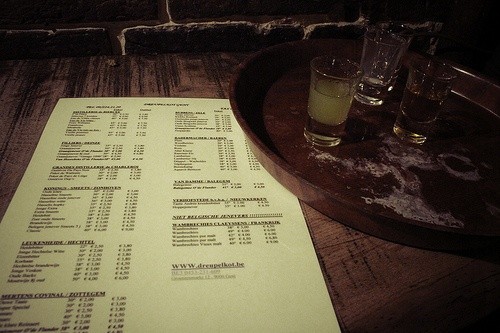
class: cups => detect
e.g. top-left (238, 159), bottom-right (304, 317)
top-left (368, 26), bottom-right (415, 92)
top-left (304, 56), bottom-right (363, 147)
top-left (354, 28), bottom-right (407, 106)
top-left (392, 55), bottom-right (458, 145)
